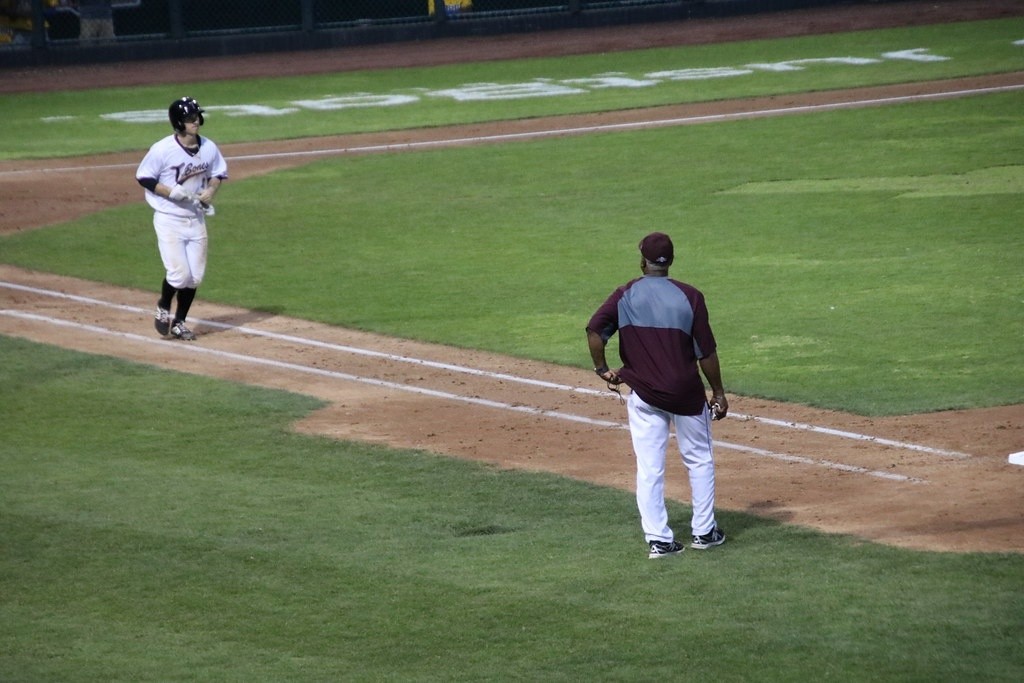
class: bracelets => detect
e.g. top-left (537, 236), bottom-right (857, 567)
top-left (713, 395), bottom-right (725, 399)
top-left (594, 365), bottom-right (609, 375)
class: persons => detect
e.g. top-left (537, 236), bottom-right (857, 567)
top-left (135, 96), bottom-right (227, 340)
top-left (0, 0), bottom-right (116, 45)
top-left (428, 0), bottom-right (474, 17)
top-left (586, 233), bottom-right (728, 560)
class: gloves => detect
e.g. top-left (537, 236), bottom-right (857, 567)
top-left (193, 195), bottom-right (215, 215)
top-left (168, 184), bottom-right (195, 202)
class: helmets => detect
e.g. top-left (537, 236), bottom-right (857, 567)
top-left (169, 97), bottom-right (204, 131)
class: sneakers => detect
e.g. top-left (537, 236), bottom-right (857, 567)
top-left (692, 527), bottom-right (726, 549)
top-left (153, 297), bottom-right (170, 335)
top-left (171, 319), bottom-right (195, 340)
top-left (649, 540), bottom-right (686, 558)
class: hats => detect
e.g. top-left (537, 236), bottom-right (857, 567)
top-left (639, 232), bottom-right (674, 263)
